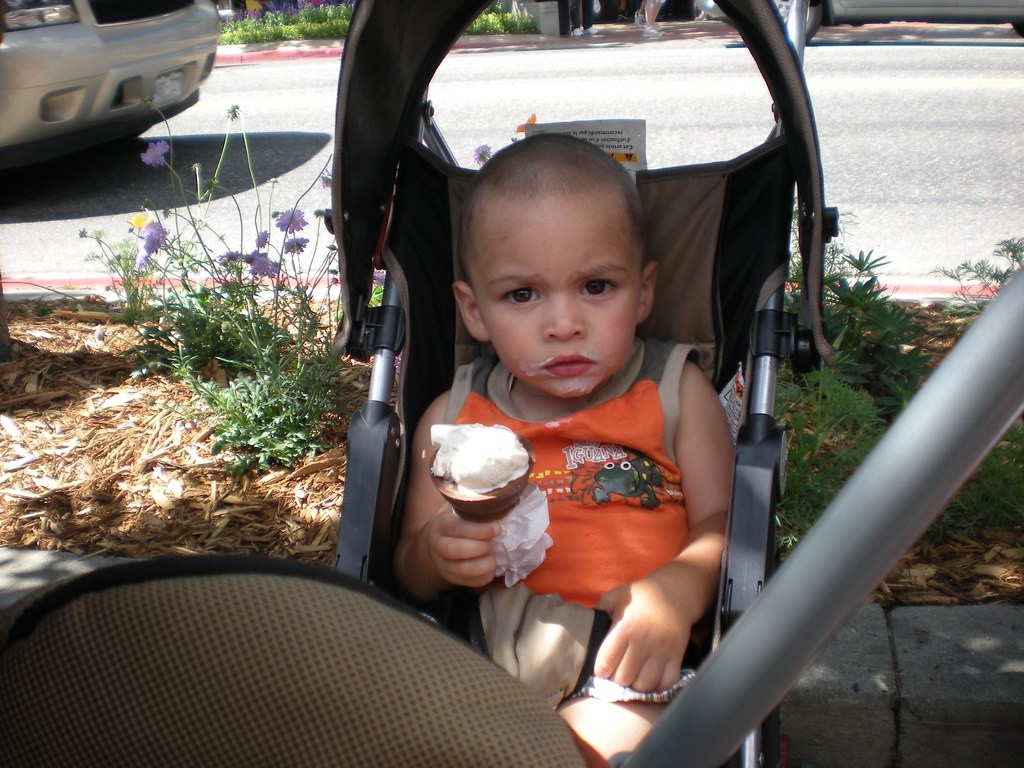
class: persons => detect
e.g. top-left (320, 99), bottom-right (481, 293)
top-left (695, 11), bottom-right (708, 21)
top-left (395, 132), bottom-right (738, 763)
top-left (635, 0), bottom-right (665, 38)
top-left (617, 0), bottom-right (630, 20)
top-left (569, 0), bottom-right (599, 36)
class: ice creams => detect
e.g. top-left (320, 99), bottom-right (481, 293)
top-left (429, 423), bottom-right (535, 523)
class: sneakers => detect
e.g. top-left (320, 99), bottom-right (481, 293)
top-left (653, 22), bottom-right (661, 32)
top-left (643, 27), bottom-right (661, 37)
top-left (583, 26), bottom-right (599, 35)
top-left (634, 10), bottom-right (645, 27)
top-left (572, 28), bottom-right (583, 36)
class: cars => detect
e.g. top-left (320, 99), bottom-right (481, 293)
top-left (0, 1), bottom-right (219, 167)
top-left (694, 0), bottom-right (1024, 44)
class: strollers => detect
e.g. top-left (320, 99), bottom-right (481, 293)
top-left (322, 0), bottom-right (838, 768)
